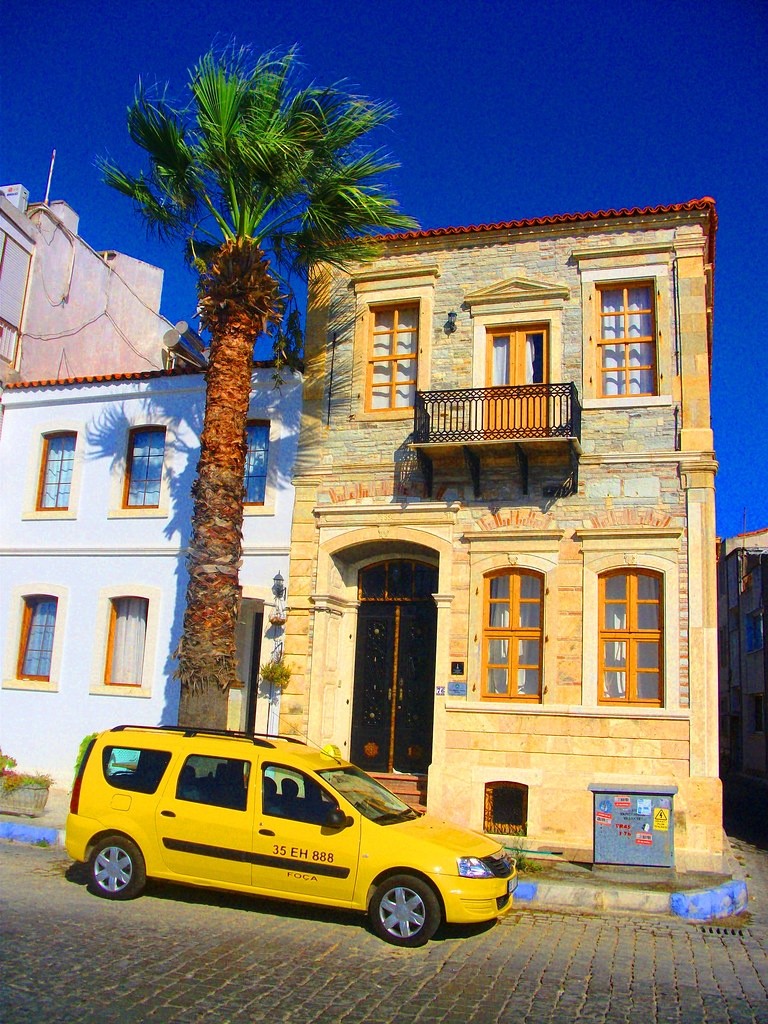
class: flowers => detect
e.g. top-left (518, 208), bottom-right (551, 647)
top-left (0, 749), bottom-right (56, 798)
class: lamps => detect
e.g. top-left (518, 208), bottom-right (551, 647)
top-left (272, 570), bottom-right (286, 602)
top-left (448, 308), bottom-right (458, 332)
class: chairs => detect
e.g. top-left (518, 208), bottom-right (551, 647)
top-left (178, 762), bottom-right (240, 808)
top-left (264, 776), bottom-right (304, 820)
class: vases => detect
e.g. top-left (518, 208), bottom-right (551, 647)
top-left (0, 785), bottom-right (49, 816)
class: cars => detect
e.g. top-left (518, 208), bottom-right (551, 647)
top-left (65, 726), bottom-right (518, 948)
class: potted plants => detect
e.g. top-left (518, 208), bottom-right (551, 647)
top-left (261, 659), bottom-right (292, 689)
